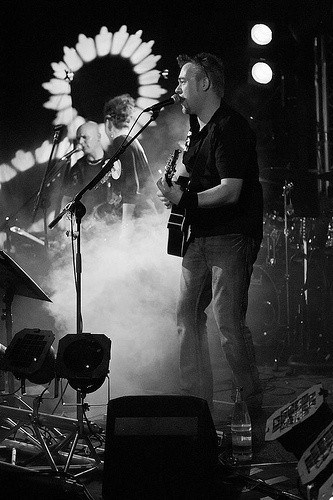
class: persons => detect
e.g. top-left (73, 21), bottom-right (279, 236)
top-left (157, 53), bottom-right (265, 421)
top-left (60, 122), bottom-right (110, 231)
top-left (100, 93), bottom-right (164, 226)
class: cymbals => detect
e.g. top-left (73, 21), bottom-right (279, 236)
top-left (259, 167), bottom-right (317, 185)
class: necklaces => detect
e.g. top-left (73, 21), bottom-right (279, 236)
top-left (88, 160), bottom-right (103, 166)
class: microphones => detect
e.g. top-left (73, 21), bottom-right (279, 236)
top-left (143, 93), bottom-right (181, 112)
top-left (60, 144), bottom-right (84, 161)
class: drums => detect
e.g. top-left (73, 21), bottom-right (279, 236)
top-left (287, 217), bottom-right (329, 251)
top-left (256, 211), bottom-right (291, 272)
top-left (245, 263), bottom-right (328, 347)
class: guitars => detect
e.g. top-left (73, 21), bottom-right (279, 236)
top-left (165, 149), bottom-right (190, 258)
top-left (60, 157), bottom-right (122, 231)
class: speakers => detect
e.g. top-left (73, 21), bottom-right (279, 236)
top-left (101, 394), bottom-right (217, 500)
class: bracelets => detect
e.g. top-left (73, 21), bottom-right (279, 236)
top-left (177, 189), bottom-right (199, 208)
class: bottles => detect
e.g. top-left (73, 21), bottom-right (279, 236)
top-left (230, 386), bottom-right (252, 461)
